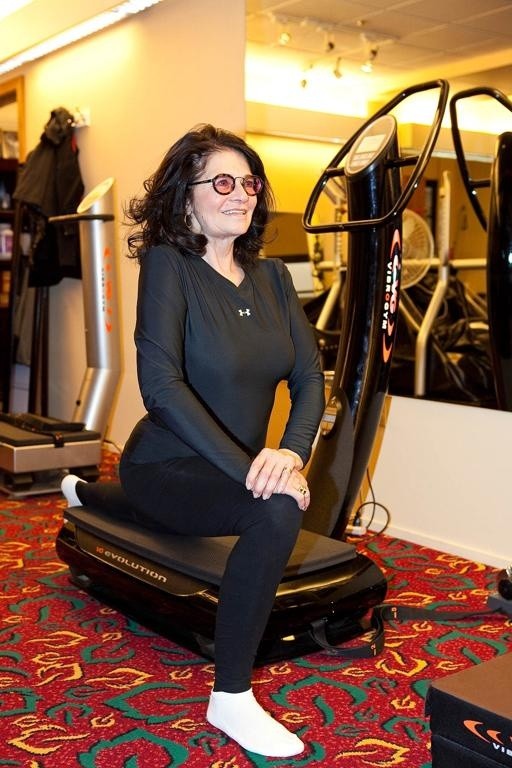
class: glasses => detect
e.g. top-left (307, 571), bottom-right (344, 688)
top-left (189, 172), bottom-right (264, 196)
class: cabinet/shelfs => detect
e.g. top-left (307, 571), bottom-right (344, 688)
top-left (0, 159), bottom-right (19, 310)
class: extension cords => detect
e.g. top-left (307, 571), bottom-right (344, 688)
top-left (346, 525), bottom-right (366, 536)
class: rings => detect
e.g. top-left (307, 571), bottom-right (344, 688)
top-left (297, 485), bottom-right (307, 496)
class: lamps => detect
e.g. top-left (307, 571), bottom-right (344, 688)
top-left (279, 19), bottom-right (398, 89)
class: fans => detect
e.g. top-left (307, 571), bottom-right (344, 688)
top-left (341, 211), bottom-right (435, 290)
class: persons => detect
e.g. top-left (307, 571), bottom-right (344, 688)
top-left (58, 121), bottom-right (327, 757)
